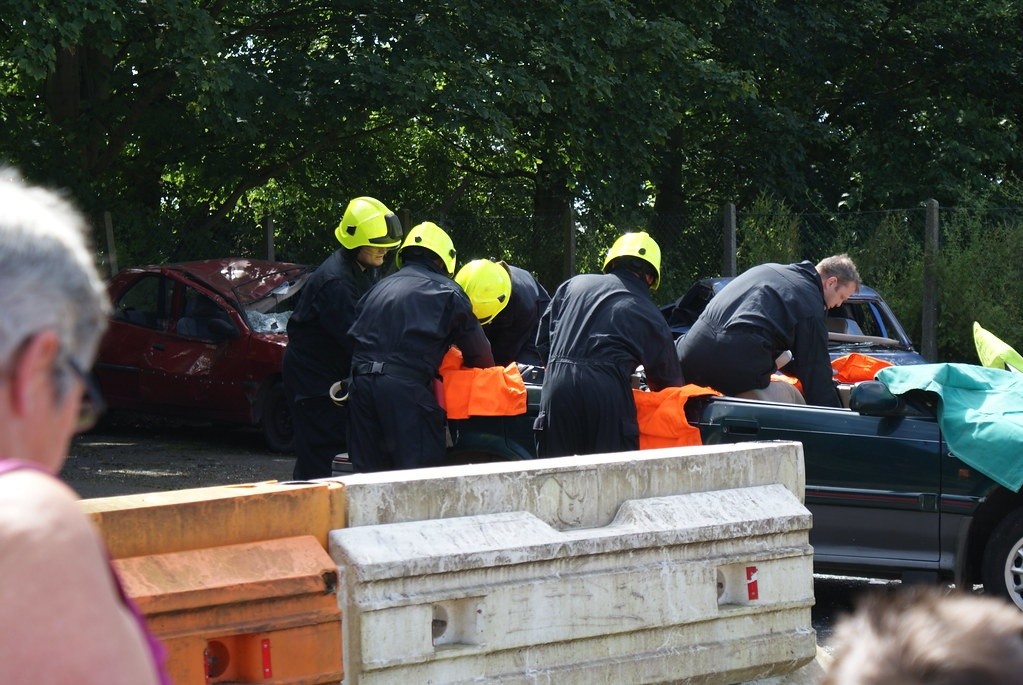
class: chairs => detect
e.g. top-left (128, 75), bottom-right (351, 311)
top-left (737, 380), bottom-right (807, 405)
top-left (177, 294), bottom-right (229, 338)
top-left (827, 317), bottom-right (864, 336)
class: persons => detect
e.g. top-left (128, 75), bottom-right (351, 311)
top-left (821, 582), bottom-right (1023, 685)
top-left (283, 196), bottom-right (402, 479)
top-left (454, 258), bottom-right (550, 369)
top-left (534, 234), bottom-right (685, 460)
top-left (344, 223), bottom-right (491, 473)
top-left (675, 254), bottom-right (862, 409)
top-left (0, 162), bottom-right (164, 685)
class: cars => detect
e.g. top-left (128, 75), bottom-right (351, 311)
top-left (440, 361), bottom-right (1022, 609)
top-left (658, 276), bottom-right (932, 376)
top-left (86, 256), bottom-right (322, 455)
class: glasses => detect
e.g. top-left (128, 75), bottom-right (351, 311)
top-left (55, 345), bottom-right (106, 434)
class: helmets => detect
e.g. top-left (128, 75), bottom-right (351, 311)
top-left (396, 222), bottom-right (456, 279)
top-left (601, 232), bottom-right (661, 291)
top-left (335, 196), bottom-right (403, 249)
top-left (453, 258), bottom-right (512, 325)
